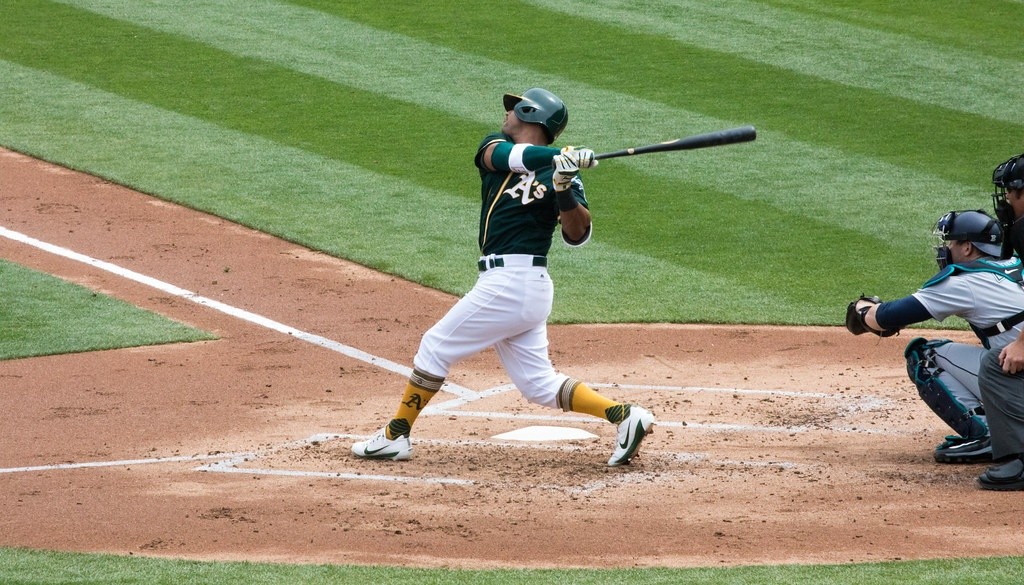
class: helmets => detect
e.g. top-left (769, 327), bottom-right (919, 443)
top-left (1006, 156), bottom-right (1024, 189)
top-left (502, 88), bottom-right (568, 142)
top-left (942, 211), bottom-right (1003, 257)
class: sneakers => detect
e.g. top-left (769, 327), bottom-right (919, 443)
top-left (350, 422), bottom-right (412, 460)
top-left (978, 457), bottom-right (1024, 490)
top-left (934, 435), bottom-right (994, 462)
top-left (607, 403), bottom-right (656, 466)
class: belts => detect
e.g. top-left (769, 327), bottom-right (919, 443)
top-left (477, 256), bottom-right (547, 271)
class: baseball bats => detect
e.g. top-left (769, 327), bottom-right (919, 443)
top-left (593, 124), bottom-right (757, 161)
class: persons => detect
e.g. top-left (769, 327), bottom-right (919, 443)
top-left (845, 154), bottom-right (1024, 491)
top-left (351, 87), bottom-right (656, 467)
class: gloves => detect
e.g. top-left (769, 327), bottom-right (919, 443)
top-left (562, 146), bottom-right (599, 168)
top-left (551, 155), bottom-right (580, 191)
